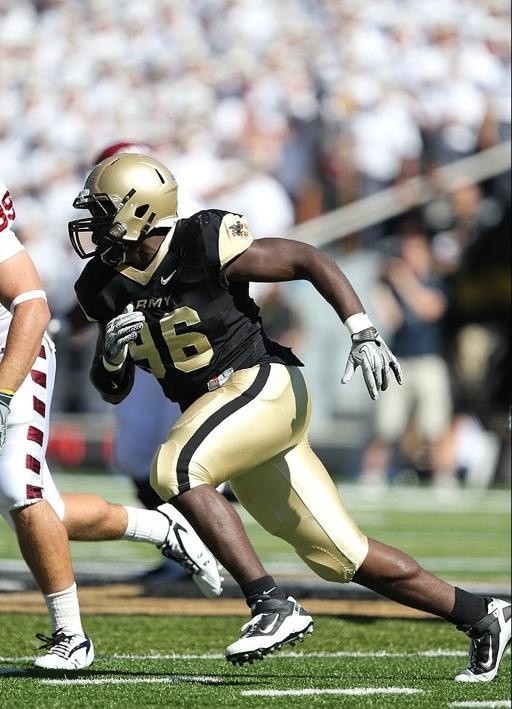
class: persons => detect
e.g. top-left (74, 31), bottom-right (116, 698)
top-left (1, 0), bottom-right (512, 511)
top-left (46, 140), bottom-right (280, 579)
top-left (0, 179), bottom-right (224, 676)
top-left (67, 152), bottom-right (509, 681)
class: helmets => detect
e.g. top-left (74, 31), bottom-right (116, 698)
top-left (68, 152), bottom-right (180, 269)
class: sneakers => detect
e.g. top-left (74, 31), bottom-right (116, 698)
top-left (453, 596), bottom-right (511, 685)
top-left (31, 625), bottom-right (94, 670)
top-left (155, 502), bottom-right (224, 599)
top-left (225, 594), bottom-right (314, 666)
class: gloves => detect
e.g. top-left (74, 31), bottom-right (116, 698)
top-left (0, 386), bottom-right (15, 449)
top-left (100, 301), bottom-right (145, 368)
top-left (339, 326), bottom-right (402, 402)
